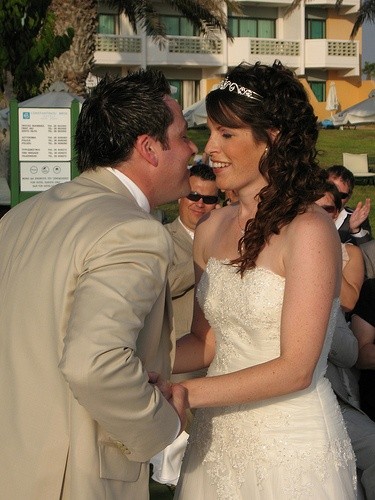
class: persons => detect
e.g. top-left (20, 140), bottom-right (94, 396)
top-left (0, 68), bottom-right (198, 500)
top-left (163, 162), bottom-right (239, 383)
top-left (313, 165), bottom-right (375, 500)
top-left (147, 59), bottom-right (357, 500)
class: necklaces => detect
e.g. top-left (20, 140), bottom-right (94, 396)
top-left (237, 204), bottom-right (245, 236)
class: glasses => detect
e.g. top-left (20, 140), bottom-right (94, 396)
top-left (321, 205), bottom-right (336, 213)
top-left (187, 193), bottom-right (218, 204)
top-left (339, 192), bottom-right (349, 200)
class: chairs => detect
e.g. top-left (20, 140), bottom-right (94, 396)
top-left (317, 118), bottom-right (357, 131)
top-left (341, 151), bottom-right (375, 185)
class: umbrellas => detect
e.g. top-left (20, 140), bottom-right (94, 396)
top-left (0, 80), bottom-right (85, 128)
top-left (182, 95), bottom-right (207, 128)
top-left (318, 89), bottom-right (375, 126)
top-left (326, 82), bottom-right (338, 116)
